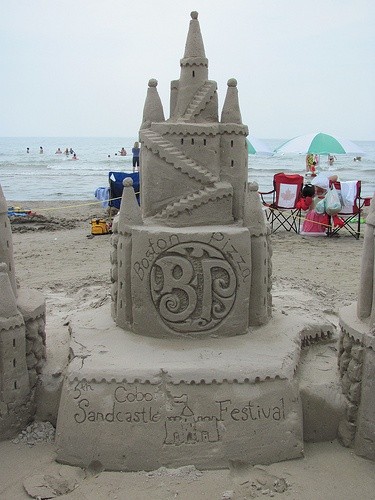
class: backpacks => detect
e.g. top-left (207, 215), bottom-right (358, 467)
top-left (91, 217), bottom-right (113, 234)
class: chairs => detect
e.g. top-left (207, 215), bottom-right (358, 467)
top-left (95, 172), bottom-right (139, 224)
top-left (259, 172), bottom-right (303, 235)
top-left (318, 180), bottom-right (372, 240)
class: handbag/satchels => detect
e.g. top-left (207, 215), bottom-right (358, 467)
top-left (301, 183), bottom-right (315, 197)
top-left (302, 209), bottom-right (325, 232)
top-left (314, 183), bottom-right (340, 216)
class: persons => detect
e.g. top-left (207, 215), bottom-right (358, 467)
top-left (132, 142), bottom-right (140, 173)
top-left (26, 144), bottom-right (363, 176)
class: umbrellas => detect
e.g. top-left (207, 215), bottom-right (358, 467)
top-left (271, 130), bottom-right (364, 176)
top-left (246, 138), bottom-right (256, 155)
